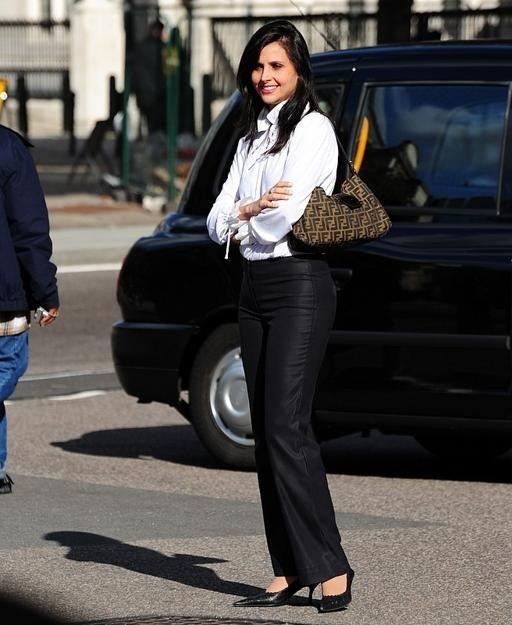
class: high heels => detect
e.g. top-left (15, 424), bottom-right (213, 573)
top-left (232, 566), bottom-right (354, 613)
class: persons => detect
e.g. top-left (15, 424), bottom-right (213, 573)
top-left (0, 79), bottom-right (61, 492)
top-left (206, 18), bottom-right (355, 612)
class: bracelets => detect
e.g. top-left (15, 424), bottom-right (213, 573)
top-left (50, 313), bottom-right (58, 319)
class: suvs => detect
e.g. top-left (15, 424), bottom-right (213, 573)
top-left (109, 36), bottom-right (512, 472)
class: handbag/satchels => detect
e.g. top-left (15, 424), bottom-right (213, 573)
top-left (284, 110), bottom-right (394, 247)
